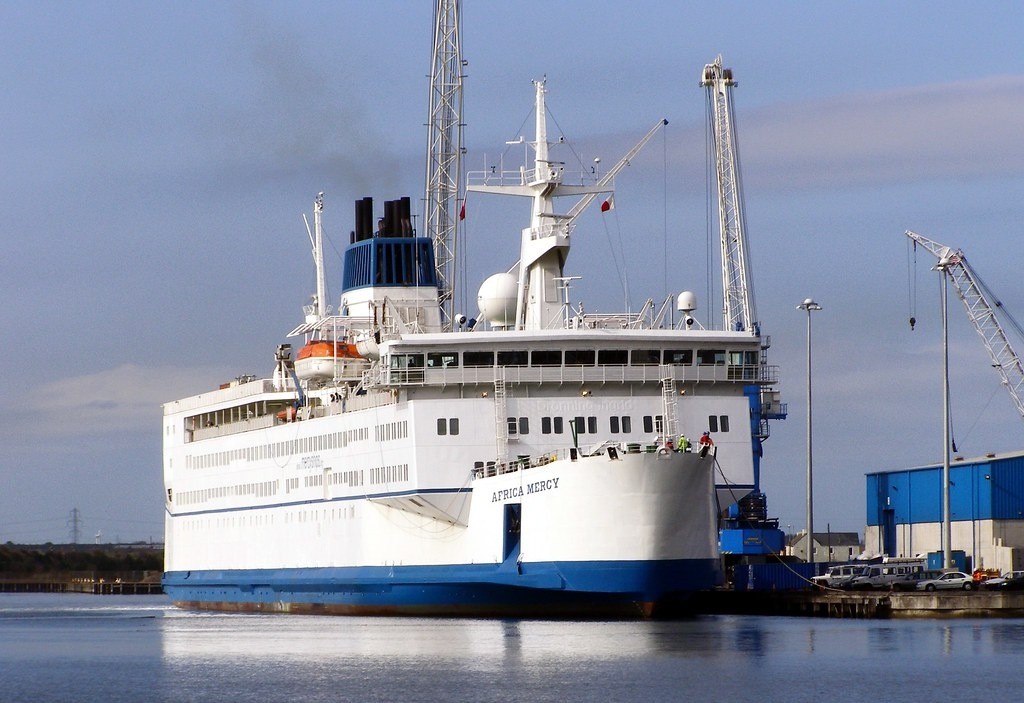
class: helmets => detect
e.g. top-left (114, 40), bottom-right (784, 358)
top-left (687, 438), bottom-right (690, 442)
top-left (668, 439), bottom-right (673, 442)
top-left (681, 434), bottom-right (684, 437)
top-left (702, 431), bottom-right (710, 435)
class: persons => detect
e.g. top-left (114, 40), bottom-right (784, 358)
top-left (699, 431), bottom-right (714, 459)
top-left (330, 393), bottom-right (341, 402)
top-left (973, 568), bottom-right (980, 591)
top-left (678, 434), bottom-right (693, 453)
top-left (207, 421), bottom-right (213, 427)
top-left (666, 439), bottom-right (677, 452)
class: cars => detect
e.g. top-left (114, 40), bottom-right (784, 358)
top-left (915, 571), bottom-right (973, 592)
top-left (839, 575), bottom-right (860, 589)
top-left (886, 568), bottom-right (943, 591)
top-left (982, 570), bottom-right (1024, 591)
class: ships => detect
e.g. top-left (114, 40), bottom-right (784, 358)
top-left (159, 0), bottom-right (785, 618)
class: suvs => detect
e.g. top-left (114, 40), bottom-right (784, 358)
top-left (810, 563), bottom-right (869, 588)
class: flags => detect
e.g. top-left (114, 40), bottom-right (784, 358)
top-left (459, 197), bottom-right (465, 220)
top-left (601, 194), bottom-right (614, 213)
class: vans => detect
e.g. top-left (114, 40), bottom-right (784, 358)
top-left (852, 562), bottom-right (926, 590)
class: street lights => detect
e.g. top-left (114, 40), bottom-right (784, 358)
top-left (796, 299), bottom-right (824, 559)
top-left (935, 258), bottom-right (958, 570)
